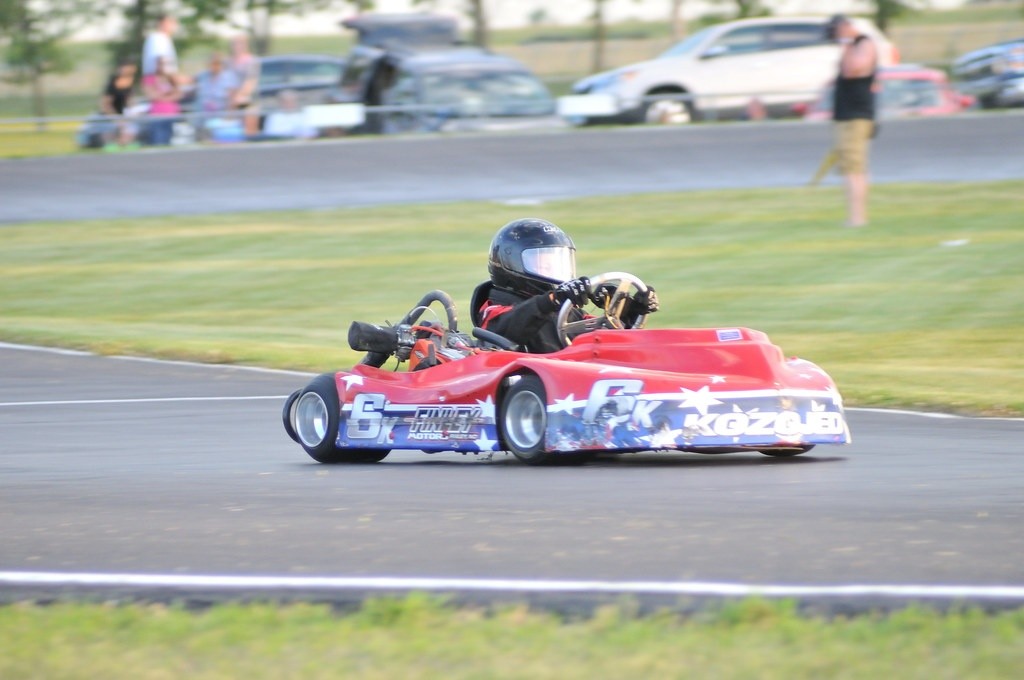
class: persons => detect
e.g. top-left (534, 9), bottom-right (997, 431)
top-left (91, 13), bottom-right (318, 151)
top-left (825, 35), bottom-right (882, 226)
top-left (478, 218), bottom-right (658, 354)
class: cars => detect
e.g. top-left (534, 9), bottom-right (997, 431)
top-left (953, 39), bottom-right (1024, 105)
top-left (189, 9), bottom-right (567, 144)
top-left (792, 64), bottom-right (978, 124)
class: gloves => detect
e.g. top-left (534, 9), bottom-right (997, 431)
top-left (631, 285), bottom-right (659, 317)
top-left (552, 276), bottom-right (591, 310)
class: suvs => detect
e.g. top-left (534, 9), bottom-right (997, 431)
top-left (554, 14), bottom-right (903, 130)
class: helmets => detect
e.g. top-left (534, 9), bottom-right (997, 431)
top-left (488, 217), bottom-right (576, 298)
